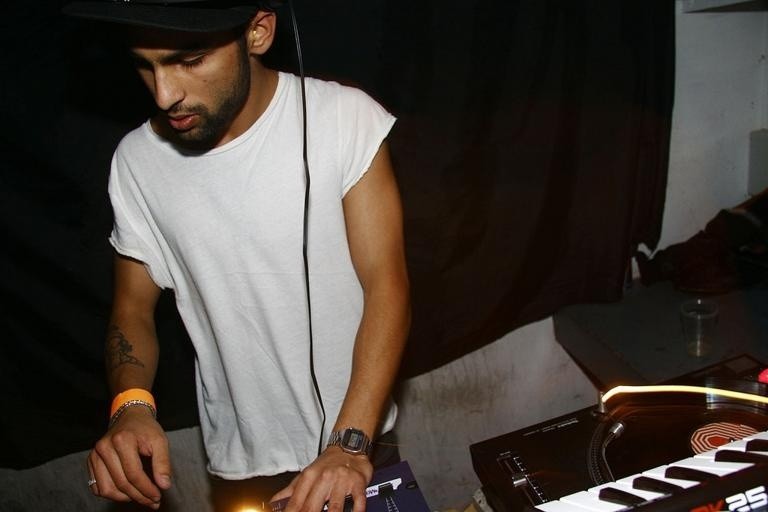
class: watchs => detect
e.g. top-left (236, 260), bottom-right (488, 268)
top-left (325, 425), bottom-right (376, 462)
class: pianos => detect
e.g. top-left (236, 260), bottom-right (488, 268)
top-left (468, 351), bottom-right (768, 512)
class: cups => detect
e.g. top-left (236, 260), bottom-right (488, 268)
top-left (680, 298), bottom-right (719, 357)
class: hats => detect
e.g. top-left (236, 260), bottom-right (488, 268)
top-left (59, 0), bottom-right (283, 34)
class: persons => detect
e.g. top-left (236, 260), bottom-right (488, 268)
top-left (86, 1), bottom-right (415, 511)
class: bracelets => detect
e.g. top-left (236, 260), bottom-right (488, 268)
top-left (108, 387), bottom-right (158, 419)
top-left (106, 399), bottom-right (158, 431)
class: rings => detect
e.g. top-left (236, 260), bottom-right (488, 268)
top-left (87, 477), bottom-right (97, 488)
